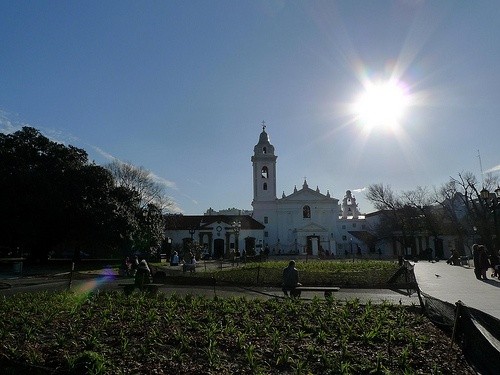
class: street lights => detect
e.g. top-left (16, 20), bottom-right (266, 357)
top-left (232, 219), bottom-right (242, 266)
top-left (481, 186), bottom-right (500, 279)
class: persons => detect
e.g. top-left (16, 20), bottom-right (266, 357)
top-left (476, 245), bottom-right (490, 281)
top-left (119, 256), bottom-right (130, 278)
top-left (427, 246), bottom-right (433, 262)
top-left (231, 245), bottom-right (271, 264)
top-left (134, 259), bottom-right (153, 284)
top-left (472, 244), bottom-right (482, 280)
top-left (282, 260), bottom-right (302, 298)
top-left (170, 251), bottom-right (180, 266)
top-left (317, 246), bottom-right (335, 260)
top-left (131, 256), bottom-right (140, 278)
top-left (182, 249), bottom-right (197, 273)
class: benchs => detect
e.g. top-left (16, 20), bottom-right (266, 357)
top-left (117, 283), bottom-right (164, 294)
top-left (282, 286), bottom-right (340, 300)
